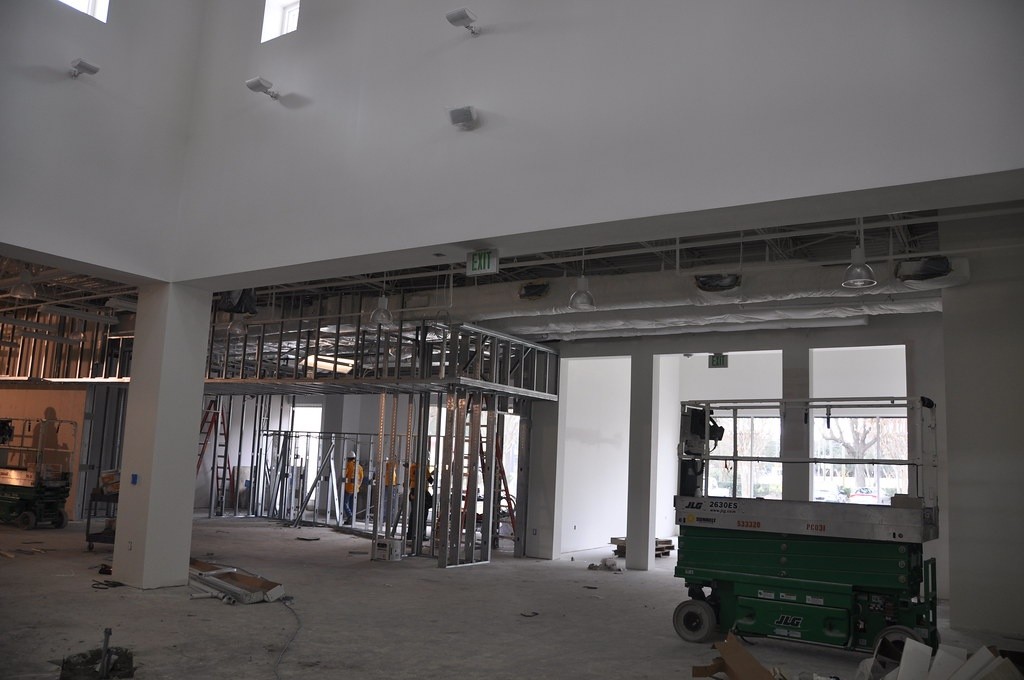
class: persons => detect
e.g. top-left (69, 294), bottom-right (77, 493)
top-left (407, 450), bottom-right (434, 542)
top-left (343, 450), bottom-right (364, 525)
top-left (381, 456), bottom-right (398, 527)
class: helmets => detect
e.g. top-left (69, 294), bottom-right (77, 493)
top-left (425, 450), bottom-right (431, 460)
top-left (346, 451), bottom-right (356, 458)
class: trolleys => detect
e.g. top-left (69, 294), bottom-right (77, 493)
top-left (85, 493), bottom-right (120, 551)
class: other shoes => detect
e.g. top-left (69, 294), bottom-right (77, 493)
top-left (344, 516), bottom-right (352, 525)
top-left (407, 533), bottom-right (429, 541)
